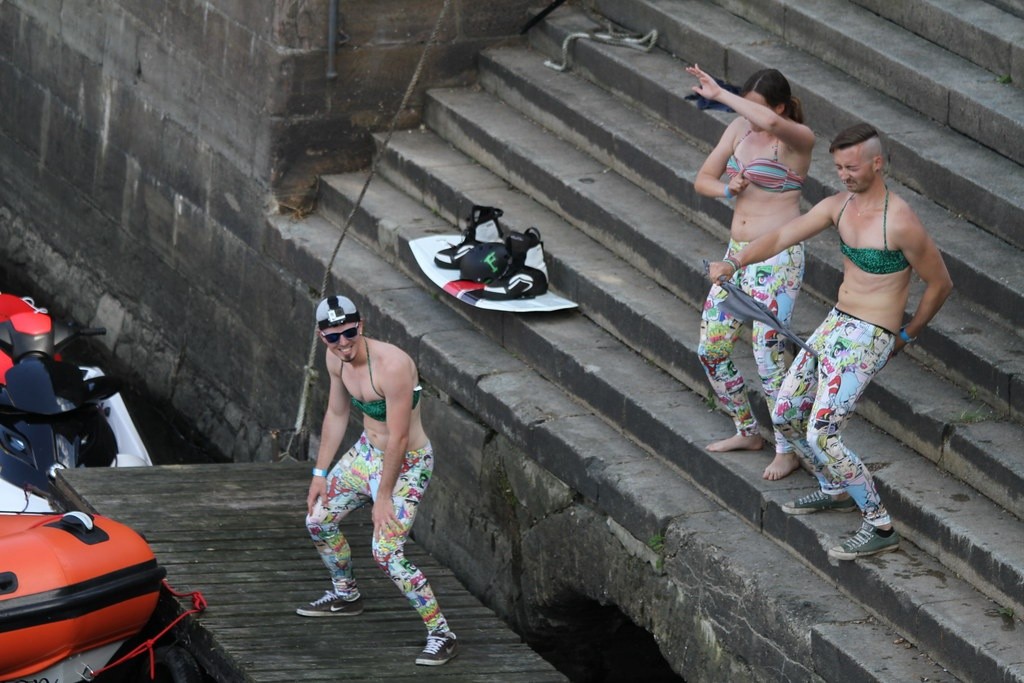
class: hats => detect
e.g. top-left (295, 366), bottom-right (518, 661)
top-left (316, 295), bottom-right (359, 329)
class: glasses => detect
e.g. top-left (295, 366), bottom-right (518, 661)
top-left (321, 323), bottom-right (359, 343)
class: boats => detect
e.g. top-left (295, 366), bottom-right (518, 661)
top-left (0, 293), bottom-right (168, 683)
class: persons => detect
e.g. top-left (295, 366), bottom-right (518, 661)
top-left (296, 294), bottom-right (459, 666)
top-left (708, 122), bottom-right (952, 560)
top-left (686, 61), bottom-right (815, 482)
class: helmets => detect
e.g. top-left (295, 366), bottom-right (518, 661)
top-left (459, 241), bottom-right (509, 283)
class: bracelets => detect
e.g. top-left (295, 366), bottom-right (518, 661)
top-left (900, 329), bottom-right (917, 343)
top-left (312, 468), bottom-right (328, 477)
top-left (723, 185), bottom-right (732, 199)
top-left (725, 256), bottom-right (740, 271)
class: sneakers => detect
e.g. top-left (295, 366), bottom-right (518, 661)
top-left (484, 227), bottom-right (549, 298)
top-left (295, 590), bottom-right (364, 617)
top-left (781, 489), bottom-right (858, 514)
top-left (435, 206), bottom-right (504, 267)
top-left (828, 521), bottom-right (901, 560)
top-left (415, 629), bottom-right (459, 667)
